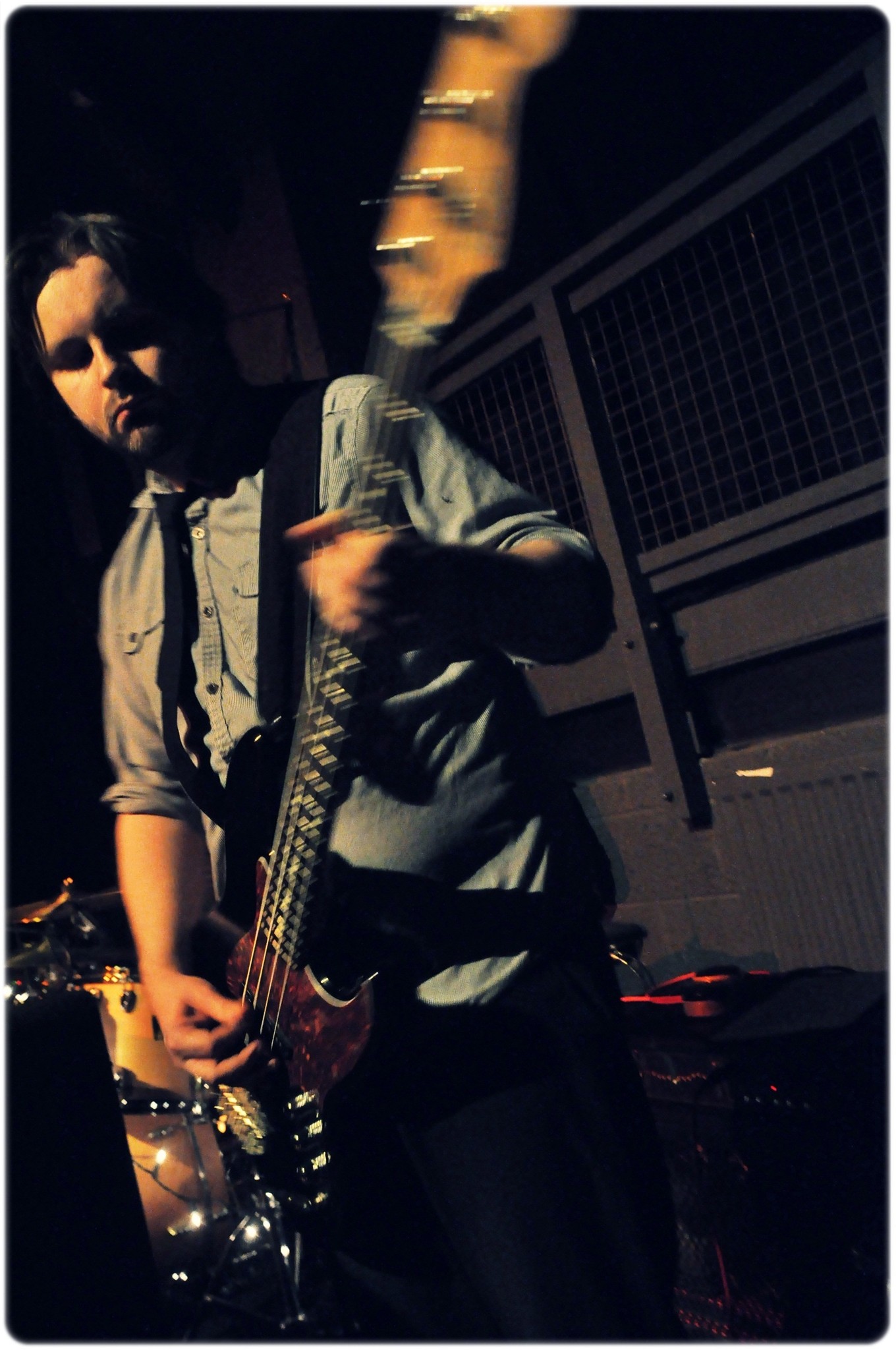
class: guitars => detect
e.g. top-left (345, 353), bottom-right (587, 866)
top-left (225, 6), bottom-right (578, 1202)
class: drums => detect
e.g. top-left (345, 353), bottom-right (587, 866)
top-left (61, 963), bottom-right (241, 1252)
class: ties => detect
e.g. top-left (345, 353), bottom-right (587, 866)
top-left (154, 495), bottom-right (226, 823)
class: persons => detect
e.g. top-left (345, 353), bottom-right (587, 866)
top-left (7, 210), bottom-right (675, 1340)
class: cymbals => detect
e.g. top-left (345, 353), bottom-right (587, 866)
top-left (7, 891), bottom-right (126, 926)
